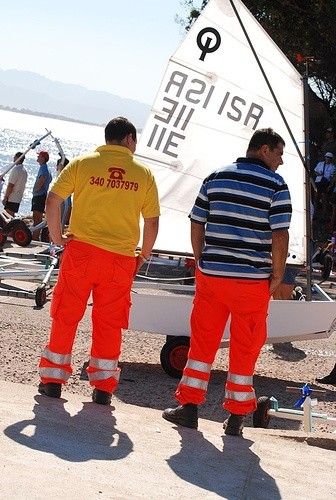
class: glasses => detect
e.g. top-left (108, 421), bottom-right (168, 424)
top-left (325, 157), bottom-right (332, 159)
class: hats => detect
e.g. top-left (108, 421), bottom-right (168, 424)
top-left (36, 151), bottom-right (49, 160)
top-left (325, 152), bottom-right (333, 158)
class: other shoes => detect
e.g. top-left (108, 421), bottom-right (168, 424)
top-left (92, 388), bottom-right (112, 405)
top-left (38, 381), bottom-right (61, 398)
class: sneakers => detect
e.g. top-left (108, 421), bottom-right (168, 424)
top-left (223, 414), bottom-right (246, 436)
top-left (162, 403), bottom-right (198, 429)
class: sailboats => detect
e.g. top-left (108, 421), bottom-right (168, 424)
top-left (93, 0), bottom-right (336, 341)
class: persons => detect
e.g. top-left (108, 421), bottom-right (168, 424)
top-left (31, 150), bottom-right (52, 241)
top-left (38, 117), bottom-right (161, 404)
top-left (162, 127), bottom-right (336, 436)
top-left (56, 152), bottom-right (71, 238)
top-left (0, 153), bottom-right (28, 216)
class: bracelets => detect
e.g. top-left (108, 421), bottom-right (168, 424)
top-left (5, 195), bottom-right (8, 199)
top-left (139, 251), bottom-right (147, 263)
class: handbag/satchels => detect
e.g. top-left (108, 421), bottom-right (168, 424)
top-left (321, 176), bottom-right (329, 188)
